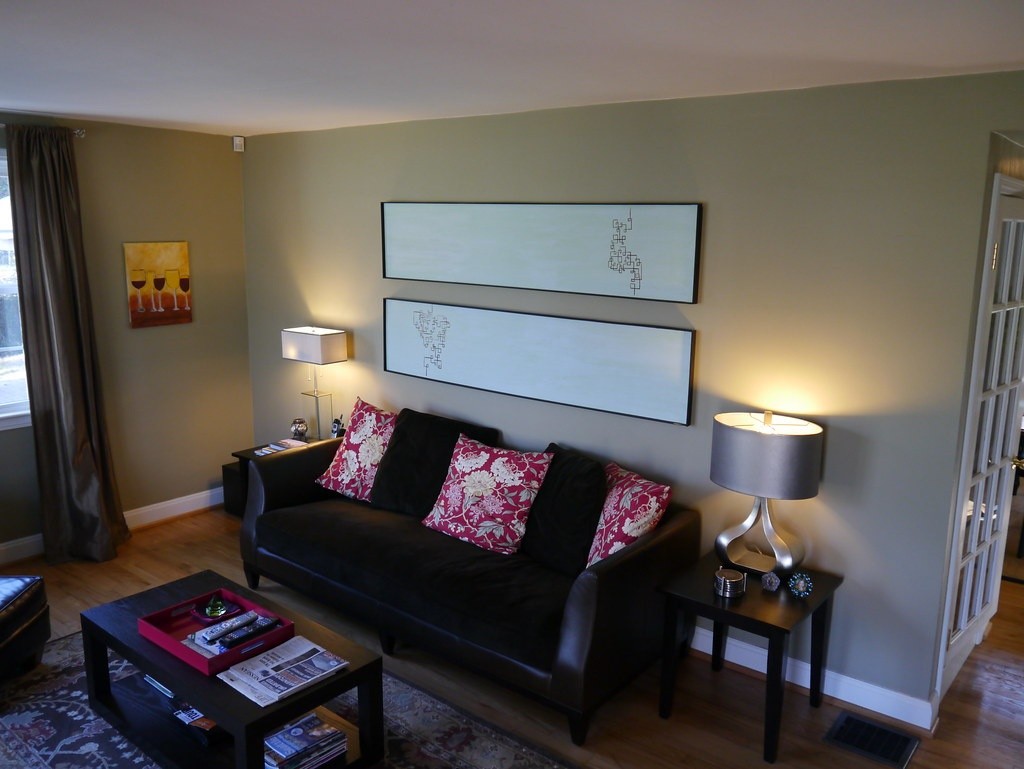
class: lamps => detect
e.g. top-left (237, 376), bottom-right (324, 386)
top-left (710, 412), bottom-right (823, 577)
top-left (281, 326), bottom-right (348, 441)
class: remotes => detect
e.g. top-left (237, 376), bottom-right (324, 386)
top-left (219, 616), bottom-right (279, 648)
top-left (202, 610), bottom-right (258, 640)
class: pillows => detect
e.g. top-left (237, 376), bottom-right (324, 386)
top-left (421, 433), bottom-right (554, 556)
top-left (586, 461), bottom-right (674, 571)
top-left (313, 397), bottom-right (397, 503)
top-left (543, 442), bottom-right (607, 577)
top-left (369, 408), bottom-right (499, 516)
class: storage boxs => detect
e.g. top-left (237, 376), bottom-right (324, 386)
top-left (138, 586), bottom-right (294, 676)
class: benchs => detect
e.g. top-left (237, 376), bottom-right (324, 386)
top-left (241, 435), bottom-right (702, 747)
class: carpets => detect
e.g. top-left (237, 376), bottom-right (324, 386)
top-left (0, 631), bottom-right (586, 769)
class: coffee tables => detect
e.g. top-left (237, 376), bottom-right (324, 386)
top-left (80, 570), bottom-right (384, 769)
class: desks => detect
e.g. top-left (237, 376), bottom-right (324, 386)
top-left (658, 549), bottom-right (844, 763)
top-left (231, 437), bottom-right (325, 521)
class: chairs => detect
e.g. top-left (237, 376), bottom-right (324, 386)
top-left (0, 575), bottom-right (51, 671)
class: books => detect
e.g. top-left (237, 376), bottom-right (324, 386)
top-left (144, 675), bottom-right (175, 698)
top-left (254, 438), bottom-right (308, 456)
top-left (263, 713), bottom-right (349, 769)
top-left (216, 636), bottom-right (350, 708)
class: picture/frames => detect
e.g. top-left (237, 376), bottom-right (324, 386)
top-left (380, 202), bottom-right (703, 304)
top-left (382, 298), bottom-right (696, 427)
top-left (789, 573), bottom-right (814, 598)
top-left (762, 572), bottom-right (780, 591)
